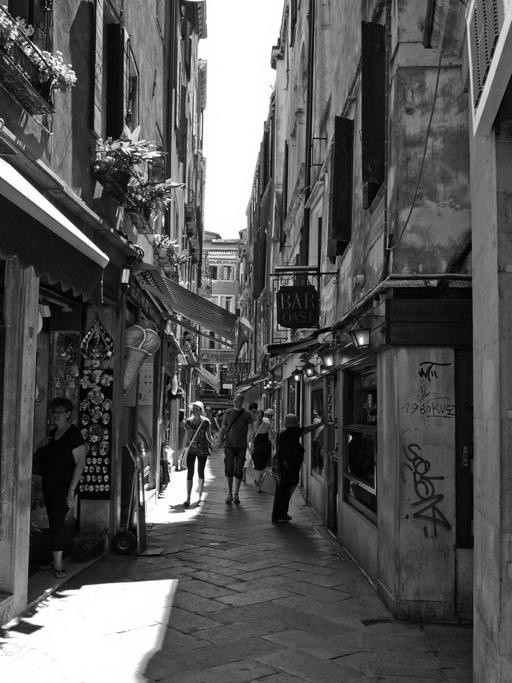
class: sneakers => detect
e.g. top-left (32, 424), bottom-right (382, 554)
top-left (271, 513), bottom-right (293, 524)
top-left (183, 500), bottom-right (202, 506)
top-left (40, 559), bottom-right (67, 579)
top-left (227, 494), bottom-right (241, 504)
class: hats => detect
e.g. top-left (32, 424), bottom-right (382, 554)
top-left (188, 400), bottom-right (204, 415)
top-left (264, 408), bottom-right (275, 416)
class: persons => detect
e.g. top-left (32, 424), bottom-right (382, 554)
top-left (241, 401), bottom-right (258, 482)
top-left (178, 399), bottom-right (221, 507)
top-left (311, 409), bottom-right (324, 471)
top-left (35, 395), bottom-right (89, 579)
top-left (271, 412), bottom-right (325, 524)
top-left (261, 408), bottom-right (276, 436)
top-left (214, 392), bottom-right (257, 504)
top-left (248, 409), bottom-right (276, 493)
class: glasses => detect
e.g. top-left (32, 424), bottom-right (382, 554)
top-left (50, 409), bottom-right (68, 415)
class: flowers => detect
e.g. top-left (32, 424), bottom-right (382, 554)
top-left (96, 120), bottom-right (189, 267)
top-left (0, 0), bottom-right (77, 95)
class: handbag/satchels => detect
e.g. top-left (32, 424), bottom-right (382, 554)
top-left (176, 447), bottom-right (189, 470)
top-left (254, 472), bottom-right (281, 496)
top-left (32, 449), bottom-right (45, 475)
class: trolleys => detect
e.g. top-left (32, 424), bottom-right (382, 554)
top-left (113, 440), bottom-right (166, 556)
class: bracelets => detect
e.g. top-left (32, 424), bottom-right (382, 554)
top-left (68, 488), bottom-right (74, 492)
